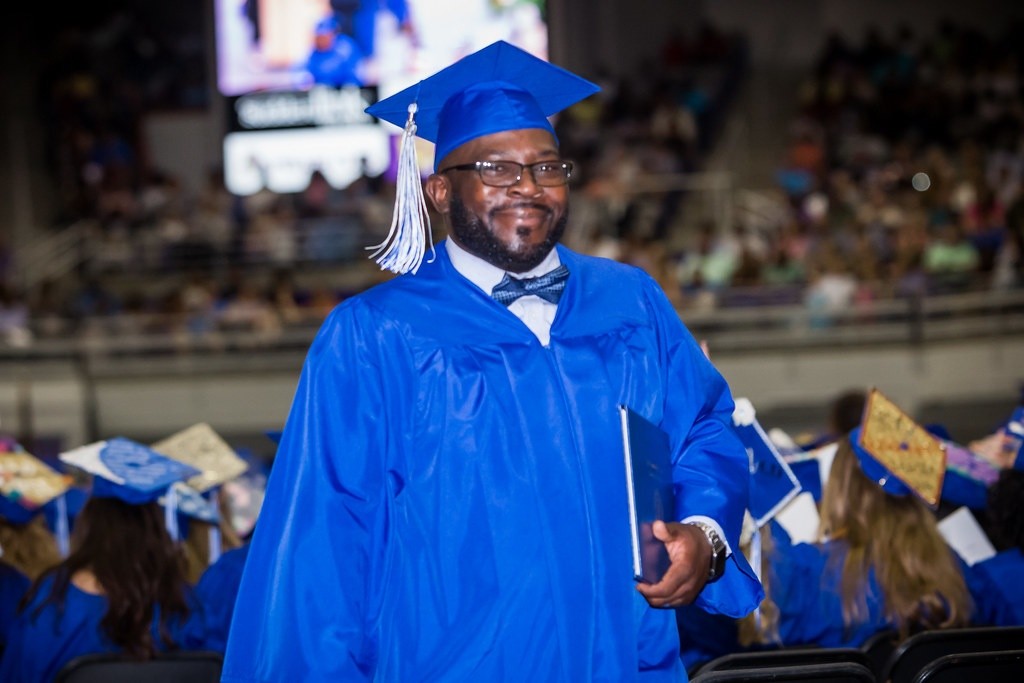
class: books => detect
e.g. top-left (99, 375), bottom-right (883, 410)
top-left (617, 405), bottom-right (675, 585)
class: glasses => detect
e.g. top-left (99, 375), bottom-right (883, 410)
top-left (440, 159), bottom-right (572, 188)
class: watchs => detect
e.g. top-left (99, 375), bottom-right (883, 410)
top-left (689, 522), bottom-right (726, 583)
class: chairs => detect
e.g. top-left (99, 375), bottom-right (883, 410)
top-left (691, 618), bottom-right (1024, 683)
top-left (49, 649), bottom-right (225, 683)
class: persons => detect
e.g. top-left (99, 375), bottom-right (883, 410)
top-left (0, 0), bottom-right (397, 356)
top-left (218, 40), bottom-right (767, 683)
top-left (0, 423), bottom-right (288, 683)
top-left (735, 386), bottom-right (1024, 649)
top-left (557, 16), bottom-right (1024, 338)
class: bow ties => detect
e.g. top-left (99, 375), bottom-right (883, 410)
top-left (492, 266), bottom-right (570, 307)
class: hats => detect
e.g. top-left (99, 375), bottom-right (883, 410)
top-left (221, 447), bottom-right (272, 538)
top-left (0, 436), bottom-right (74, 560)
top-left (848, 386), bottom-right (948, 508)
top-left (364, 40), bottom-right (604, 275)
top-left (149, 422), bottom-right (251, 565)
top-left (58, 436), bottom-right (202, 539)
